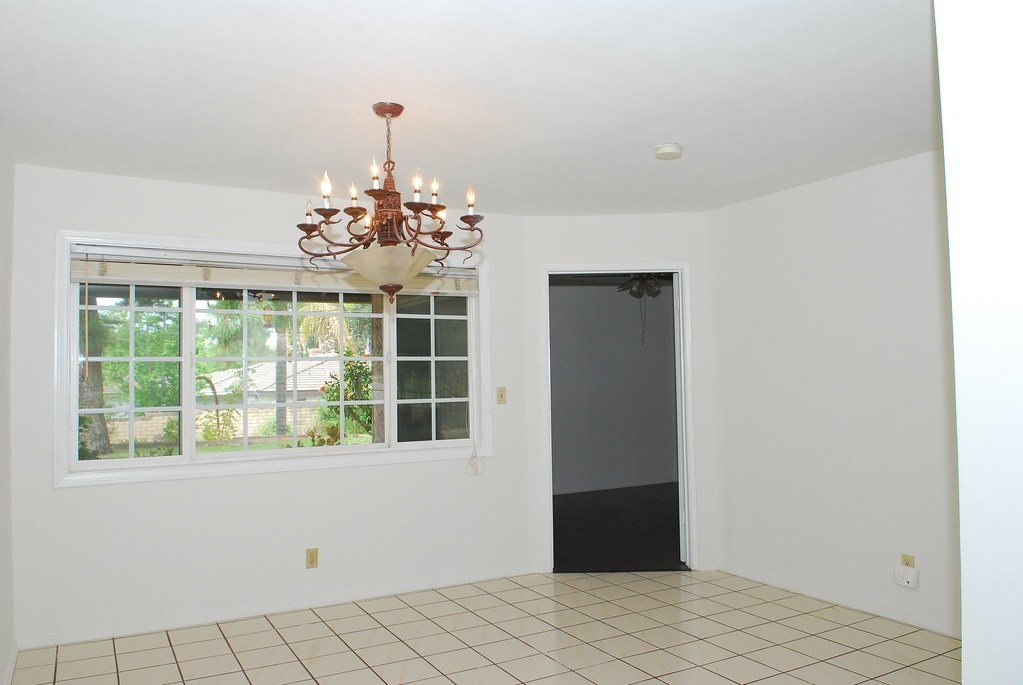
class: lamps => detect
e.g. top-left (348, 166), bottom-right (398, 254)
top-left (295, 100), bottom-right (485, 301)
top-left (615, 273), bottom-right (666, 347)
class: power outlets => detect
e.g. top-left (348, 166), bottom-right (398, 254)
top-left (902, 553), bottom-right (914, 568)
top-left (306, 547), bottom-right (319, 569)
top-left (893, 565), bottom-right (919, 588)
top-left (498, 387), bottom-right (507, 404)
